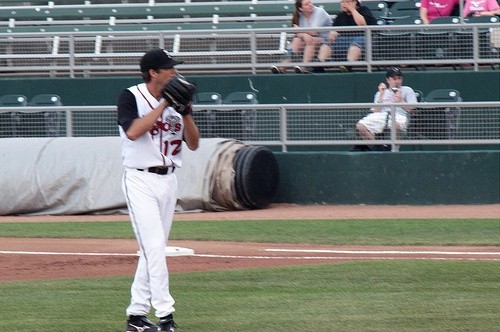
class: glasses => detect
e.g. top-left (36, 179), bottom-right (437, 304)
top-left (300, 0), bottom-right (311, 7)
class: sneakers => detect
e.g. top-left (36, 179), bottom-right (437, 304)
top-left (126, 315), bottom-right (161, 332)
top-left (157, 318), bottom-right (178, 332)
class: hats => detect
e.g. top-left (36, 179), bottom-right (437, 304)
top-left (141, 49), bottom-right (183, 72)
top-left (385, 67), bottom-right (402, 76)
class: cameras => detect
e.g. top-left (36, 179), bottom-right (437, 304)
top-left (392, 87), bottom-right (398, 94)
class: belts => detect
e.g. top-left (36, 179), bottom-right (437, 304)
top-left (137, 166), bottom-right (175, 175)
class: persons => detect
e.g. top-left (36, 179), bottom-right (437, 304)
top-left (354, 67), bottom-right (418, 153)
top-left (419, 0), bottom-right (462, 24)
top-left (117, 48), bottom-right (200, 332)
top-left (311, 0), bottom-right (377, 73)
top-left (270, 0), bottom-right (334, 74)
top-left (461, 0), bottom-right (500, 16)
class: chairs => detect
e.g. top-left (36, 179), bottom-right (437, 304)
top-left (1, 0), bottom-right (500, 70)
top-left (422, 89), bottom-right (462, 150)
top-left (407, 91), bottom-right (423, 149)
top-left (185, 92), bottom-right (222, 138)
top-left (0, 95), bottom-right (28, 135)
top-left (225, 93), bottom-right (260, 148)
top-left (31, 95), bottom-right (61, 136)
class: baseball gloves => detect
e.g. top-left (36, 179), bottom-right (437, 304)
top-left (160, 75), bottom-right (196, 119)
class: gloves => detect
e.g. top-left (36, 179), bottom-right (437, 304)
top-left (161, 74), bottom-right (197, 115)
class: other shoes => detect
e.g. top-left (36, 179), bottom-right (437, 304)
top-left (313, 66), bottom-right (325, 73)
top-left (339, 65), bottom-right (352, 72)
top-left (271, 66), bottom-right (283, 74)
top-left (294, 66), bottom-right (303, 73)
